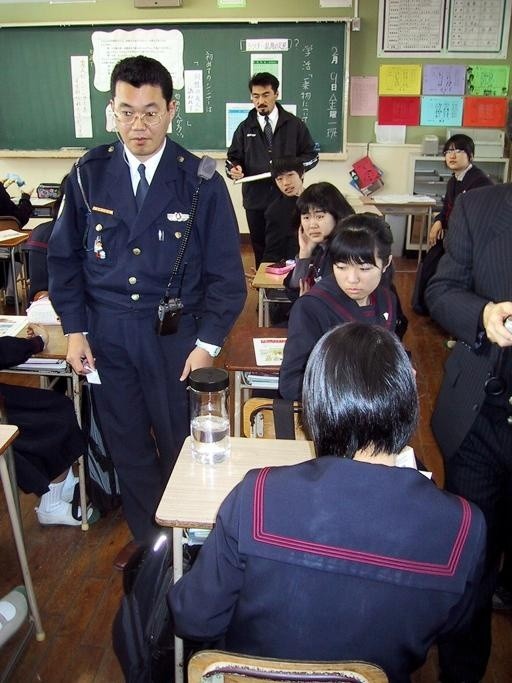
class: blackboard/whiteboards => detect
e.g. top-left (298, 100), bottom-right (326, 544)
top-left (0, 17), bottom-right (351, 162)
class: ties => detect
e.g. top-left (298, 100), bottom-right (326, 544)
top-left (264, 115), bottom-right (274, 150)
top-left (136, 163), bottom-right (150, 213)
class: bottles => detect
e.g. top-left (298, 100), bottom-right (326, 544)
top-left (186, 364), bottom-right (234, 465)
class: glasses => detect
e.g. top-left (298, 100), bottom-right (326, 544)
top-left (113, 107), bottom-right (161, 126)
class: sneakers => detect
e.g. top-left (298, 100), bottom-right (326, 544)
top-left (63, 475), bottom-right (80, 502)
top-left (0, 585), bottom-right (30, 648)
top-left (36, 504), bottom-right (100, 527)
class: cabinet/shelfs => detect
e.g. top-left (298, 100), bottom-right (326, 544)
top-left (402, 152), bottom-right (509, 259)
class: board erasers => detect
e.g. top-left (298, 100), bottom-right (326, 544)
top-left (61, 147), bottom-right (87, 150)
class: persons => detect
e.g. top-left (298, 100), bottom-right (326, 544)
top-left (2, 56), bottom-right (512, 683)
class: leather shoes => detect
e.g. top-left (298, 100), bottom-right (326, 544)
top-left (113, 538), bottom-right (146, 572)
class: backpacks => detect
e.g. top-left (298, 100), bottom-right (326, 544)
top-left (80, 380), bottom-right (123, 513)
top-left (411, 239), bottom-right (445, 317)
top-left (111, 527), bottom-right (194, 683)
